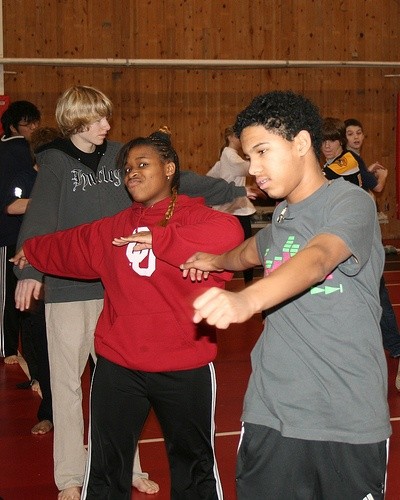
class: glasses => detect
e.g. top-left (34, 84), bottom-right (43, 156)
top-left (18, 120), bottom-right (39, 128)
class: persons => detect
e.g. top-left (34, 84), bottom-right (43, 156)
top-left (205, 117), bottom-right (400, 359)
top-left (12, 85), bottom-right (265, 500)
top-left (8, 131), bottom-right (245, 500)
top-left (179, 89), bottom-right (393, 500)
top-left (0, 100), bottom-right (64, 435)
